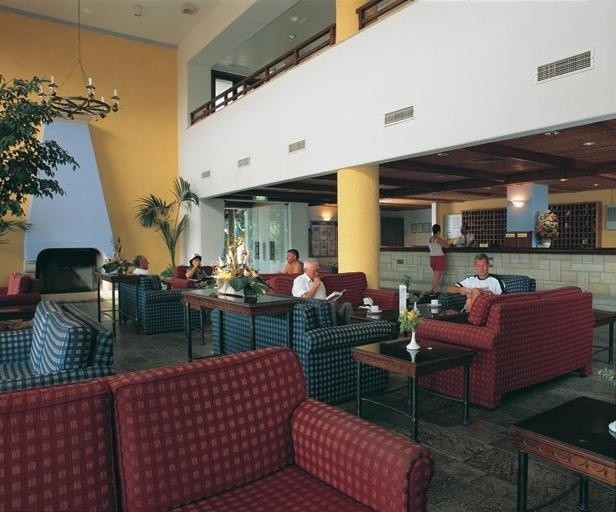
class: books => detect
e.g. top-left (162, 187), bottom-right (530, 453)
top-left (324, 289), bottom-right (348, 303)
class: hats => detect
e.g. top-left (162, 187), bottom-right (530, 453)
top-left (190, 253), bottom-right (201, 263)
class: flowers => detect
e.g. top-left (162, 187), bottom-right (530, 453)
top-left (534, 209), bottom-right (561, 240)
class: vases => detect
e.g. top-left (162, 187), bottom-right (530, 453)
top-left (537, 238), bottom-right (550, 248)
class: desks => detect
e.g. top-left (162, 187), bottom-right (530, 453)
top-left (507, 395), bottom-right (616, 511)
top-left (592, 308), bottom-right (616, 367)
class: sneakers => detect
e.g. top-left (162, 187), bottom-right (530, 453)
top-left (430, 289), bottom-right (441, 294)
top-left (430, 293), bottom-right (440, 297)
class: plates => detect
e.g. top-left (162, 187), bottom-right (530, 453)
top-left (367, 310), bottom-right (382, 315)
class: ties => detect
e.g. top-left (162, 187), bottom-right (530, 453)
top-left (465, 237), bottom-right (467, 245)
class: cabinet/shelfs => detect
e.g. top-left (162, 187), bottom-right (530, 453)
top-left (461, 207), bottom-right (506, 249)
top-left (549, 201), bottom-right (602, 249)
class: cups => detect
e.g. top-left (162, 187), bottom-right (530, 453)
top-left (431, 309), bottom-right (438, 314)
top-left (431, 299), bottom-right (438, 306)
top-left (369, 305), bottom-right (379, 312)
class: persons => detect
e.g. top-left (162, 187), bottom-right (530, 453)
top-left (282, 249), bottom-right (304, 275)
top-left (185, 254), bottom-right (208, 289)
top-left (131, 255), bottom-right (152, 275)
top-left (291, 258), bottom-right (353, 322)
top-left (426, 224), bottom-right (450, 296)
top-left (447, 254), bottom-right (505, 314)
top-left (454, 224), bottom-right (477, 248)
